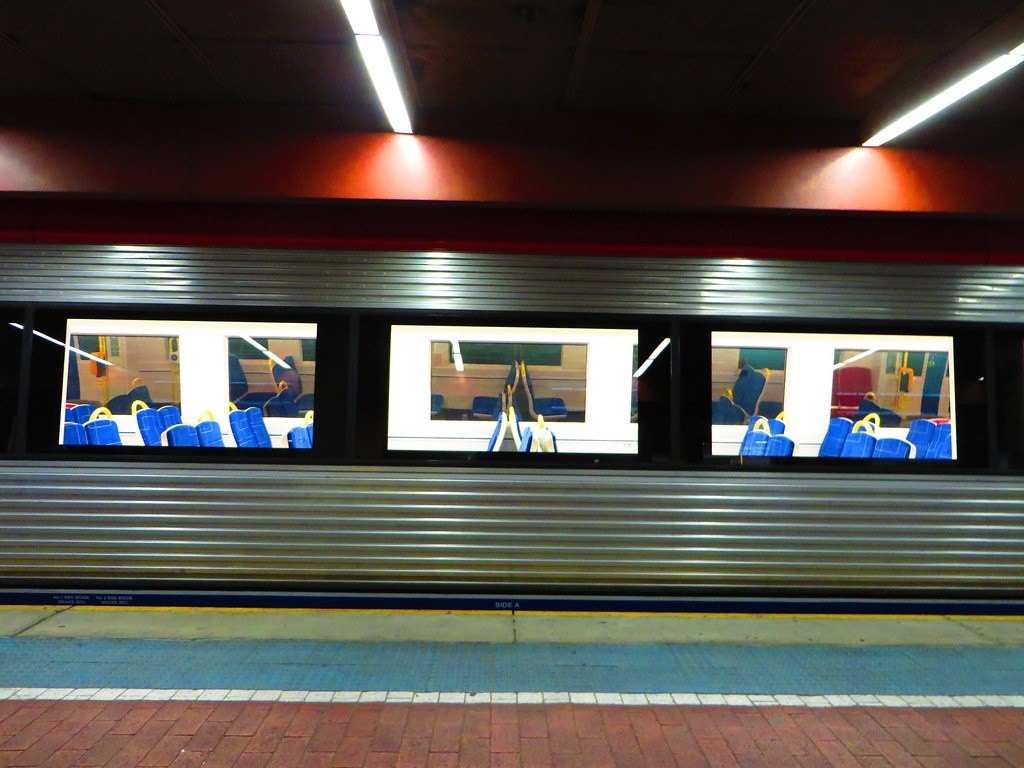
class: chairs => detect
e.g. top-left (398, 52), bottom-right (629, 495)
top-left (68, 355), bottom-right (314, 449)
top-left (712, 364), bottom-right (952, 460)
top-left (431, 344), bottom-right (567, 452)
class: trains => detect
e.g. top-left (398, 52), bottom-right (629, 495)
top-left (2, 237), bottom-right (1022, 607)
